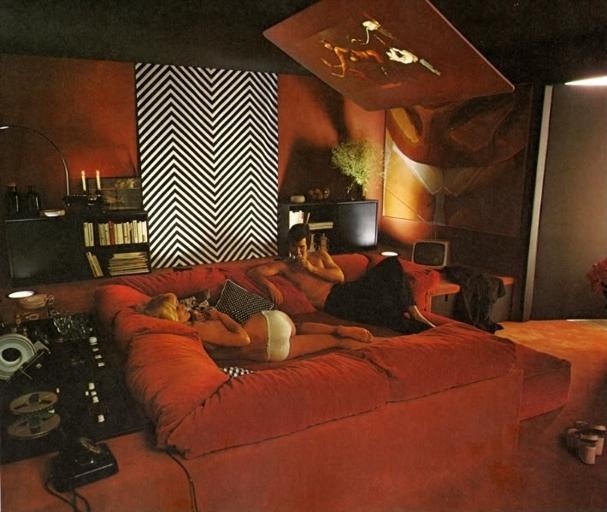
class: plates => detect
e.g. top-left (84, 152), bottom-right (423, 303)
top-left (8, 291), bottom-right (33, 299)
top-left (40, 209), bottom-right (65, 217)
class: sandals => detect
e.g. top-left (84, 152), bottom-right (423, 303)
top-left (575, 420), bottom-right (606, 456)
top-left (566, 427), bottom-right (599, 465)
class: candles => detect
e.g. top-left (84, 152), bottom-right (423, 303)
top-left (81, 170), bottom-right (101, 192)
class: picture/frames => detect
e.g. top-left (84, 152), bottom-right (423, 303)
top-left (381, 81), bottom-right (534, 240)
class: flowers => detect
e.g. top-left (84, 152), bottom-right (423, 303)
top-left (587, 259), bottom-right (607, 298)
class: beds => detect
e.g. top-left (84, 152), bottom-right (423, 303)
top-left (93, 251), bottom-right (519, 460)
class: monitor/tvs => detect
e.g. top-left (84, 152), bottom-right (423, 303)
top-left (411, 239), bottom-right (451, 270)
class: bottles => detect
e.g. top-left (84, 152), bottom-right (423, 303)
top-left (7, 183), bottom-right (42, 220)
top-left (290, 188), bottom-right (331, 203)
top-left (567, 420), bottom-right (606, 465)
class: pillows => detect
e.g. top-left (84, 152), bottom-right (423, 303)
top-left (214, 278), bottom-right (275, 325)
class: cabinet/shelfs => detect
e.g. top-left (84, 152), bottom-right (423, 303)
top-left (78, 210), bottom-right (152, 279)
top-left (279, 196), bottom-right (334, 255)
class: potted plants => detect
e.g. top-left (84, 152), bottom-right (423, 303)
top-left (327, 132), bottom-right (386, 249)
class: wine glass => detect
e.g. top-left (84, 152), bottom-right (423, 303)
top-left (54, 313), bottom-right (73, 343)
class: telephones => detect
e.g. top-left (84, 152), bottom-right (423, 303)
top-left (48, 420), bottom-right (118, 493)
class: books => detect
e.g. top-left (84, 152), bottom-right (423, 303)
top-left (81, 217), bottom-right (151, 279)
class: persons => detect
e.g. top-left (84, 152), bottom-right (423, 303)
top-left (243, 221), bottom-right (436, 335)
top-left (359, 17), bottom-right (441, 77)
top-left (129, 292), bottom-right (374, 364)
top-left (320, 38), bottom-right (394, 82)
